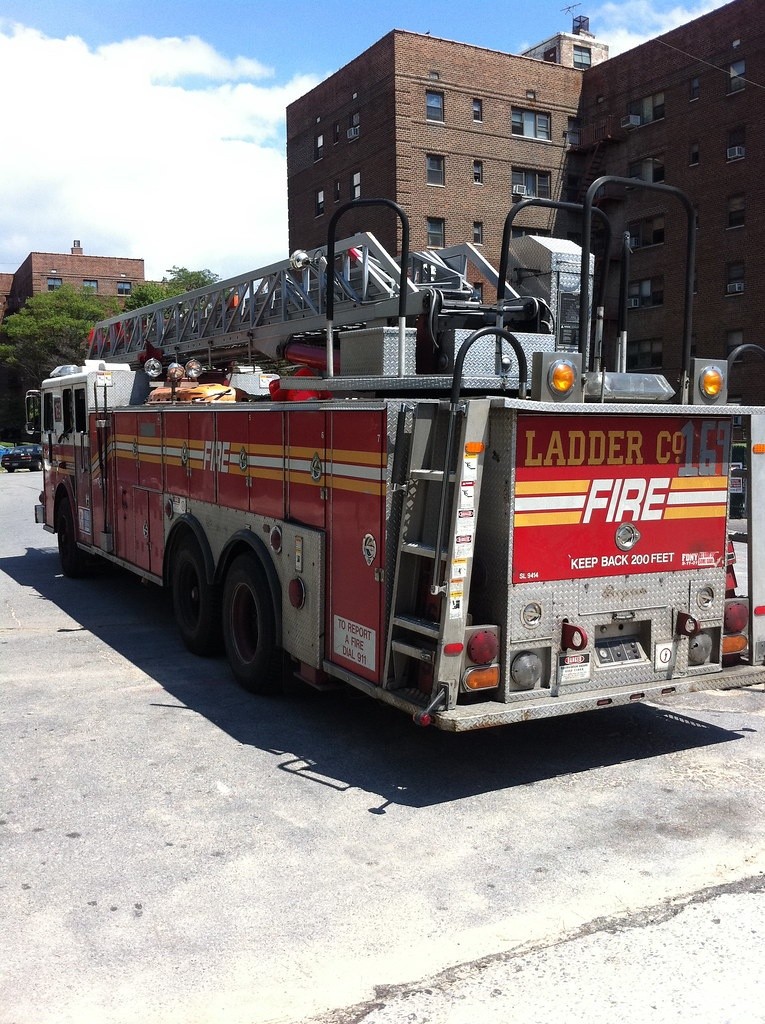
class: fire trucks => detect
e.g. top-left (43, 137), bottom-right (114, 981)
top-left (25, 177), bottom-right (765, 731)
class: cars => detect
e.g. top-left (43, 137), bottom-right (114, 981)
top-left (1, 443), bottom-right (42, 472)
